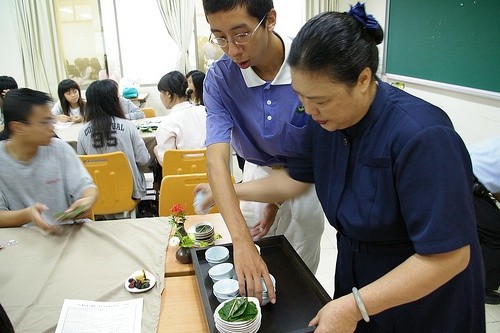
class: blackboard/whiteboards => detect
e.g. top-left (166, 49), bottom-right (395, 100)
top-left (380, 0), bottom-right (500, 102)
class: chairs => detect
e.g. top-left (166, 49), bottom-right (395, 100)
top-left (158, 173), bottom-right (241, 215)
top-left (76, 152), bottom-right (141, 221)
top-left (161, 148), bottom-right (208, 178)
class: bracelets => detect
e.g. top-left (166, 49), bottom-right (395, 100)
top-left (352, 286), bottom-right (371, 322)
top-left (274, 202), bottom-right (281, 209)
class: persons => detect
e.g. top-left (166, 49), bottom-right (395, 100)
top-left (151, 70), bottom-right (209, 218)
top-left (0, 87), bottom-right (99, 229)
top-left (50, 78), bottom-right (89, 123)
top-left (75, 79), bottom-right (151, 200)
top-left (0, 75), bottom-right (60, 142)
top-left (191, 0), bottom-right (487, 333)
top-left (202, 0), bottom-right (325, 308)
top-left (186, 70), bottom-right (207, 107)
top-left (101, 79), bottom-right (146, 123)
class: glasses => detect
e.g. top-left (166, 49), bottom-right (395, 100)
top-left (208, 15), bottom-right (266, 48)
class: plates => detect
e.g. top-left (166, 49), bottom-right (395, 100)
top-left (125, 270), bottom-right (157, 293)
top-left (213, 296), bottom-right (261, 333)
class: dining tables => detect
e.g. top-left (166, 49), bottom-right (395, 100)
top-left (0, 212), bottom-right (232, 333)
top-left (53, 115), bottom-right (168, 166)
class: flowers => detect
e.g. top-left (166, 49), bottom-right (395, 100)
top-left (170, 202), bottom-right (223, 249)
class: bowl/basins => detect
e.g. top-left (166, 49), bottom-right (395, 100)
top-left (193, 225), bottom-right (216, 248)
top-left (205, 244), bottom-right (275, 305)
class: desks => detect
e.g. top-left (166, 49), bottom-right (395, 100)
top-left (121, 91), bottom-right (150, 108)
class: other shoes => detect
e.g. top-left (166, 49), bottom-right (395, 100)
top-left (484, 291), bottom-right (499, 304)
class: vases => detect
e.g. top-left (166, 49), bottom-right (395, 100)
top-left (175, 235), bottom-right (193, 264)
